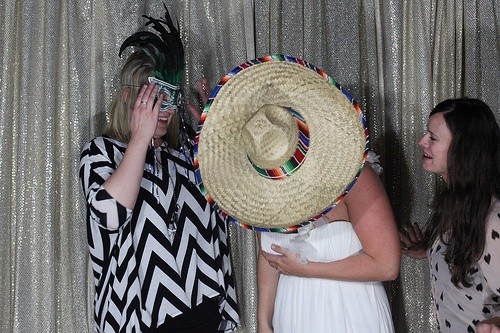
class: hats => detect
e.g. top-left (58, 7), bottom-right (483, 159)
top-left (194, 54), bottom-right (370, 233)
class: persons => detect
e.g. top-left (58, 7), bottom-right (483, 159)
top-left (258, 151), bottom-right (400, 333)
top-left (78, 51), bottom-right (242, 333)
top-left (398, 95), bottom-right (500, 333)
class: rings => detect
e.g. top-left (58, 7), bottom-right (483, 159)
top-left (141, 101), bottom-right (147, 103)
